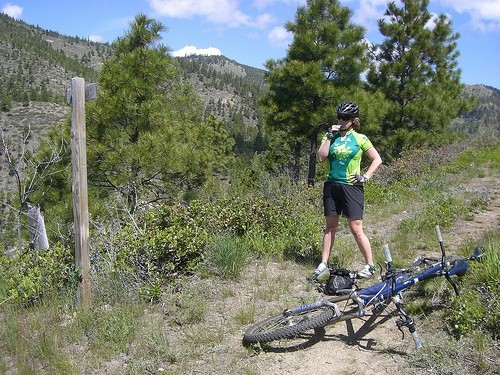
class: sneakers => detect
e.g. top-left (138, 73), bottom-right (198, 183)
top-left (315, 263), bottom-right (328, 274)
top-left (358, 264), bottom-right (375, 277)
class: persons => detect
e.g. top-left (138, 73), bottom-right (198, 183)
top-left (313, 101), bottom-right (382, 278)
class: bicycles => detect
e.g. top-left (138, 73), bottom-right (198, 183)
top-left (243, 224), bottom-right (486, 352)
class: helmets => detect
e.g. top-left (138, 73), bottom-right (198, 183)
top-left (336, 102), bottom-right (359, 116)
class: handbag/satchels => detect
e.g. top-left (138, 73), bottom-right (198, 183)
top-left (324, 268), bottom-right (354, 295)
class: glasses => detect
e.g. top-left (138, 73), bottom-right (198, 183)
top-left (337, 114), bottom-right (353, 121)
